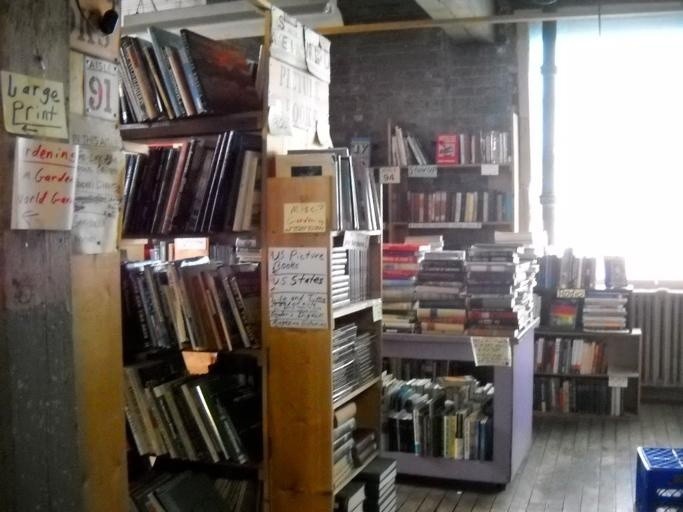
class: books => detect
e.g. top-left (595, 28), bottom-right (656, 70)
top-left (117, 27), bottom-right (267, 512)
top-left (270, 147), bottom-right (399, 511)
top-left (381, 111), bottom-right (538, 461)
top-left (534, 249), bottom-right (633, 416)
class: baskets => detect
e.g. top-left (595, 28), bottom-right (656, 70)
top-left (634, 447), bottom-right (683, 512)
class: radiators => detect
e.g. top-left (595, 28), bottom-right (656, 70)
top-left (631, 289), bottom-right (683, 389)
